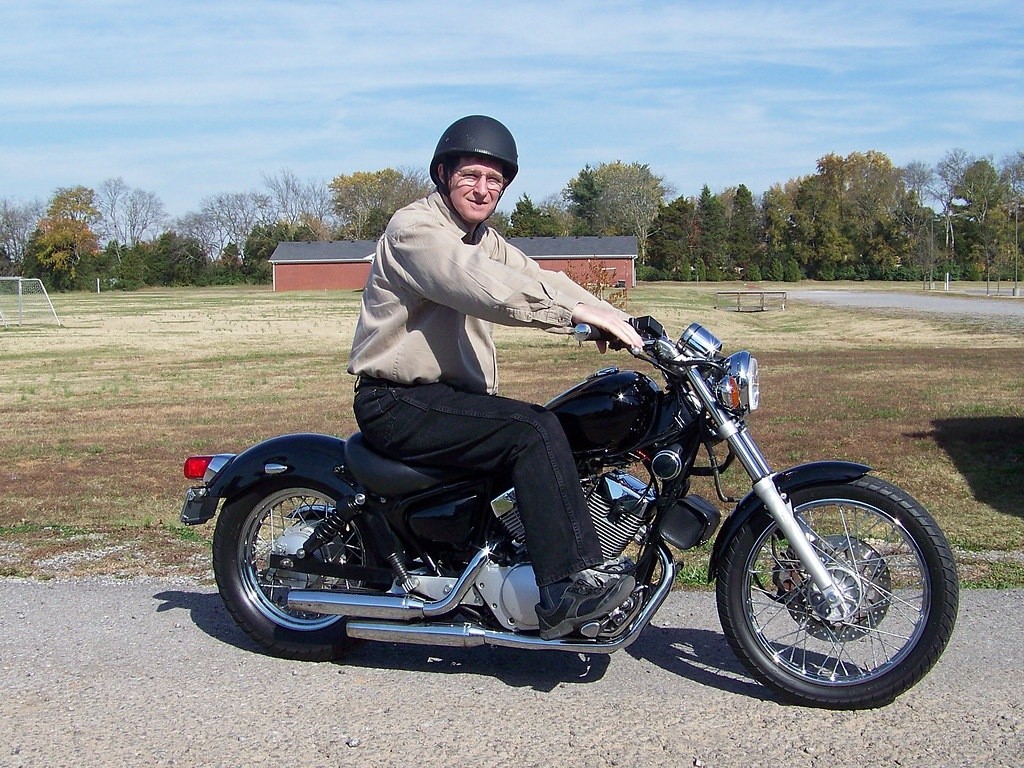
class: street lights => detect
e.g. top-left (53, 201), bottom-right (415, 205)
top-left (1012, 204), bottom-right (1024, 296)
top-left (929, 218), bottom-right (940, 291)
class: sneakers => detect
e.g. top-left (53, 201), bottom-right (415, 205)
top-left (535, 576), bottom-right (634, 642)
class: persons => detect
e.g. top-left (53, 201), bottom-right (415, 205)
top-left (347, 116), bottom-right (644, 641)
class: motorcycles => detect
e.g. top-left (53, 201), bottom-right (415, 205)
top-left (178, 316), bottom-right (961, 710)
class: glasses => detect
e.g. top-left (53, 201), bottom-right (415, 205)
top-left (451, 169), bottom-right (507, 192)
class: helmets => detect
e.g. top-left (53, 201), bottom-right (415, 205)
top-left (429, 115), bottom-right (519, 195)
